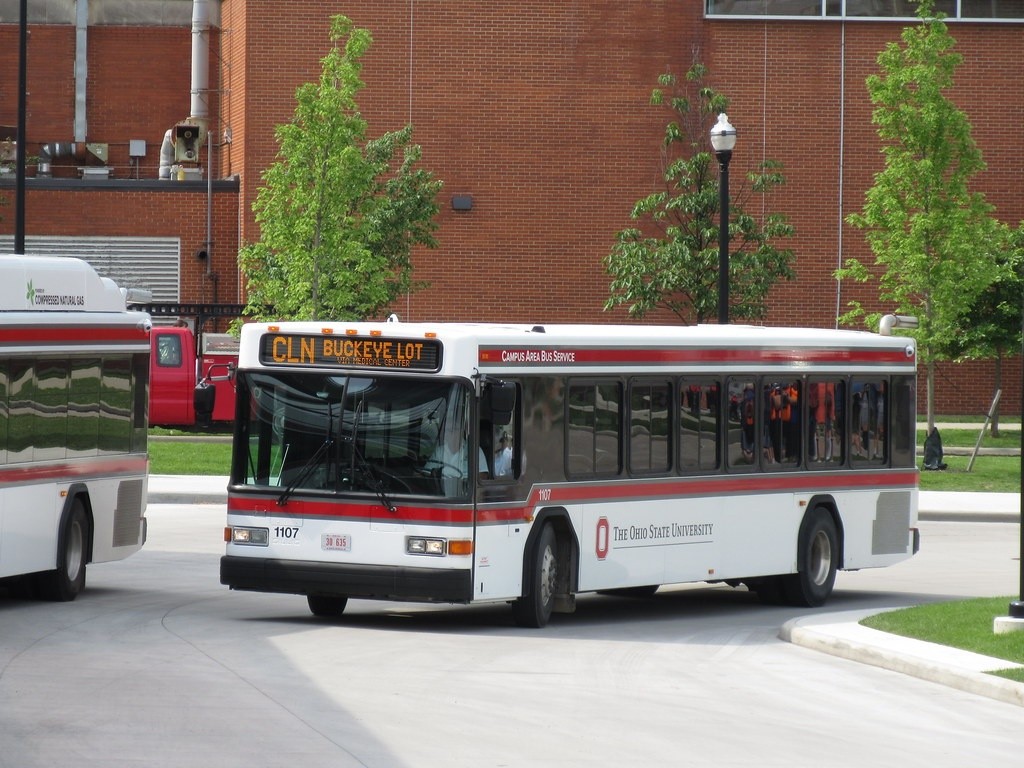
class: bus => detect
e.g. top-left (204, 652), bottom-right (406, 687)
top-left (0, 252), bottom-right (151, 605)
top-left (218, 311), bottom-right (920, 630)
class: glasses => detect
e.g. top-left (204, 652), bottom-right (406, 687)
top-left (442, 428), bottom-right (459, 433)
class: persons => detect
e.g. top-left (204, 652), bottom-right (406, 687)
top-left (489, 433), bottom-right (528, 477)
top-left (737, 380), bottom-right (883, 463)
top-left (410, 412), bottom-right (489, 500)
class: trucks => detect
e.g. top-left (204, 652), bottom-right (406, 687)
top-left (148, 325), bottom-right (265, 427)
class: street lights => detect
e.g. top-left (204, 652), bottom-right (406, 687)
top-left (709, 114), bottom-right (737, 328)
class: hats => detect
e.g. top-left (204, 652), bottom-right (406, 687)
top-left (773, 383), bottom-right (779, 389)
top-left (499, 431), bottom-right (508, 441)
